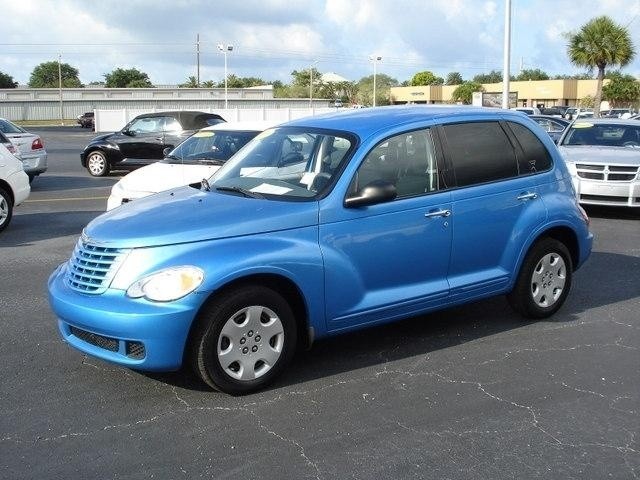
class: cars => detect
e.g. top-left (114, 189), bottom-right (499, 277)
top-left (104, 117), bottom-right (390, 213)
top-left (45, 99), bottom-right (595, 398)
top-left (79, 110), bottom-right (249, 177)
top-left (0, 117), bottom-right (49, 184)
top-left (77, 111), bottom-right (96, 131)
top-left (502, 100), bottom-right (640, 217)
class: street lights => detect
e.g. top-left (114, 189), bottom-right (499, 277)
top-left (218, 46), bottom-right (234, 109)
top-left (370, 56), bottom-right (382, 108)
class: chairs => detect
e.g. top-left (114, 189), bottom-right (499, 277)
top-left (394, 158), bottom-right (430, 196)
top-left (327, 149), bottom-right (360, 195)
top-left (619, 128), bottom-right (639, 145)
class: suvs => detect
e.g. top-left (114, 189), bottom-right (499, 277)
top-left (0, 129), bottom-right (32, 233)
